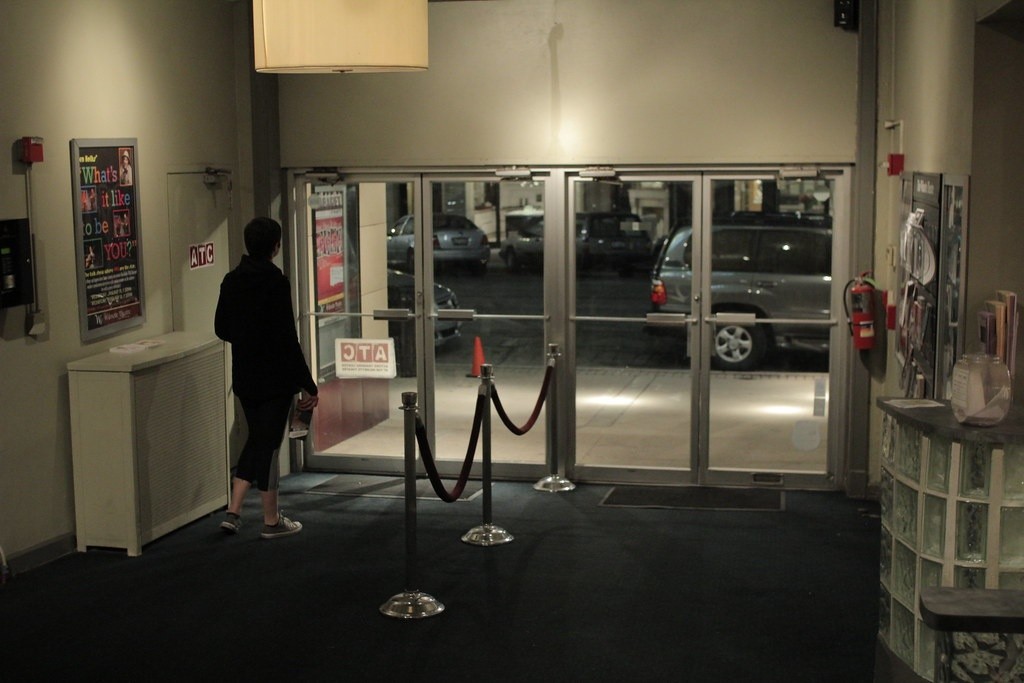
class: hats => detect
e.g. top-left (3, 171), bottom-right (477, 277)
top-left (122, 150), bottom-right (130, 161)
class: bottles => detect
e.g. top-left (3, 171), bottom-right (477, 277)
top-left (951, 352), bottom-right (1012, 427)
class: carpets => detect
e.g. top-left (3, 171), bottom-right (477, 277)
top-left (598, 485), bottom-right (786, 511)
top-left (304, 472), bottom-right (495, 501)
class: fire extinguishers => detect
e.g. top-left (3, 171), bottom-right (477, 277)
top-left (842, 271), bottom-right (881, 351)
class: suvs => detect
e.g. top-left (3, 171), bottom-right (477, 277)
top-left (646, 210), bottom-right (833, 373)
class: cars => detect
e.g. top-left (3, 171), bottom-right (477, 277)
top-left (500, 209), bottom-right (652, 274)
top-left (388, 268), bottom-right (461, 354)
top-left (387, 214), bottom-right (491, 276)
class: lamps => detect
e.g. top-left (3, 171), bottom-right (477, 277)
top-left (252, 0), bottom-right (429, 72)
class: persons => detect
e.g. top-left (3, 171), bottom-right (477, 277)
top-left (215, 217), bottom-right (319, 538)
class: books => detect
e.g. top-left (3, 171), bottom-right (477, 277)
top-left (977, 311), bottom-right (996, 357)
top-left (986, 301), bottom-right (1006, 366)
top-left (289, 399), bottom-right (311, 441)
top-left (996, 289), bottom-right (1017, 379)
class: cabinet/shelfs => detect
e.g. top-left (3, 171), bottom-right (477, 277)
top-left (66, 330), bottom-right (232, 557)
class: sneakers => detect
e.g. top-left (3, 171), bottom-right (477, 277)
top-left (219, 512), bottom-right (243, 537)
top-left (260, 513), bottom-right (303, 539)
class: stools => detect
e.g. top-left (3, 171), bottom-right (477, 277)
top-left (920, 585), bottom-right (1024, 683)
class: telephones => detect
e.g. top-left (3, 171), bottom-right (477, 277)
top-left (0, 218), bottom-right (33, 309)
